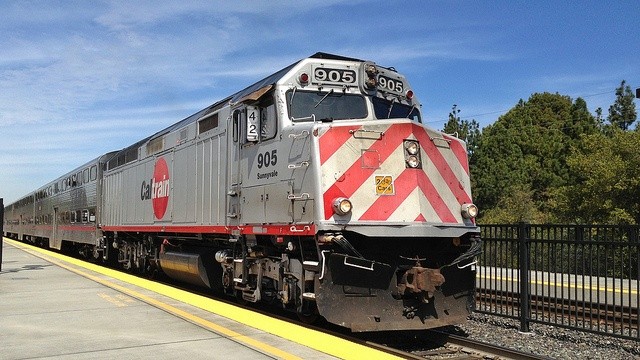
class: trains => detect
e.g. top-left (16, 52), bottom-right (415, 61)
top-left (3, 52), bottom-right (483, 351)
